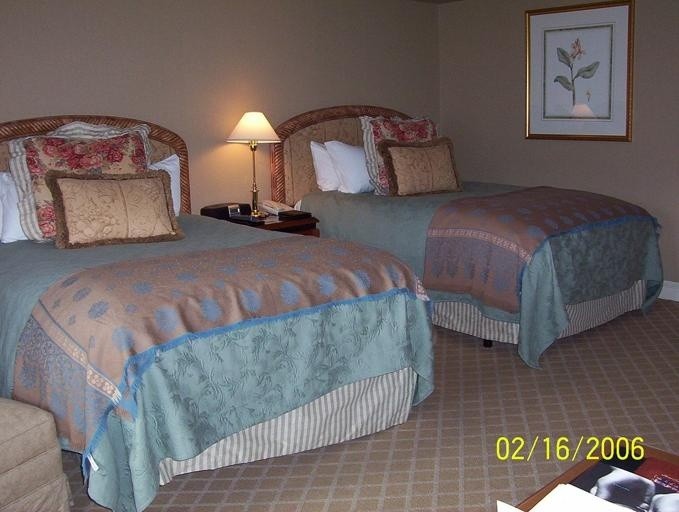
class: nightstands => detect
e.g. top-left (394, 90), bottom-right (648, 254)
top-left (215, 205), bottom-right (322, 238)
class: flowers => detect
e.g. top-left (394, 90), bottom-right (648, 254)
top-left (553, 38), bottom-right (600, 105)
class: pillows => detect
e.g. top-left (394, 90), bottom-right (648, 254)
top-left (377, 133), bottom-right (464, 197)
top-left (308, 140), bottom-right (338, 191)
top-left (15, 122), bottom-right (152, 245)
top-left (359, 113), bottom-right (440, 197)
top-left (41, 164), bottom-right (186, 251)
top-left (1, 171), bottom-right (25, 244)
top-left (323, 136), bottom-right (373, 193)
top-left (152, 153), bottom-right (181, 217)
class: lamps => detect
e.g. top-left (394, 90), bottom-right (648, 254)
top-left (227, 111), bottom-right (281, 219)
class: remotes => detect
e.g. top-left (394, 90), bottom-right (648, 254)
top-left (232, 215), bottom-right (265, 226)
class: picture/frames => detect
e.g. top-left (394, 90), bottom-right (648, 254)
top-left (523, 1), bottom-right (636, 143)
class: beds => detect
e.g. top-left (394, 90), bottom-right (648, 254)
top-left (269, 105), bottom-right (663, 372)
top-left (0, 110), bottom-right (436, 512)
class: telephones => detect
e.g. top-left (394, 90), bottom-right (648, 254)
top-left (262, 200), bottom-right (294, 216)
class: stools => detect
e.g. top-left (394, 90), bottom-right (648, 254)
top-left (0, 395), bottom-right (77, 512)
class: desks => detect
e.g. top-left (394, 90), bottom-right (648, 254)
top-left (512, 432), bottom-right (678, 512)
